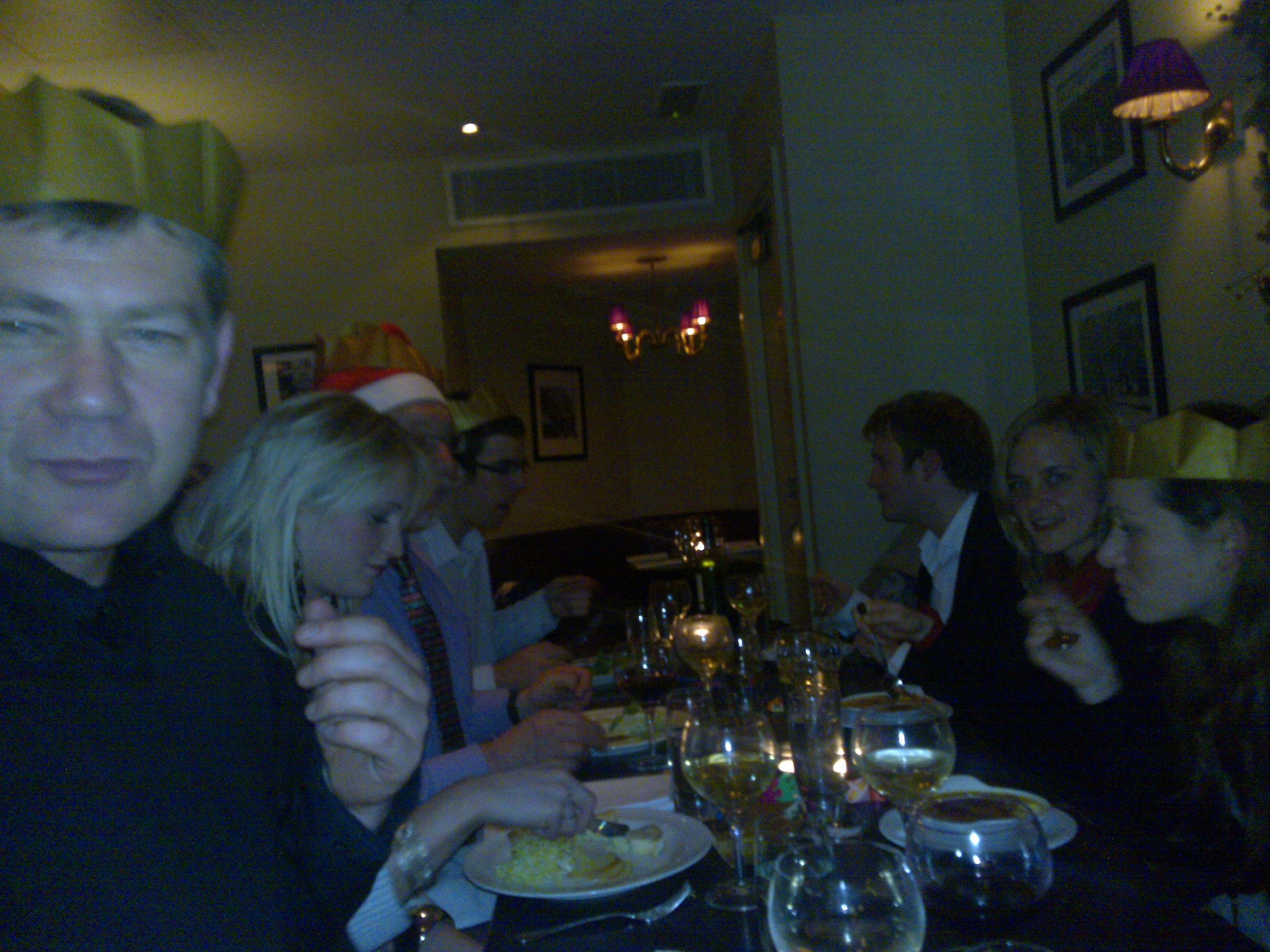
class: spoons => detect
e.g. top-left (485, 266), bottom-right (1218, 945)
top-left (1043, 611), bottom-right (1080, 650)
top-left (857, 602), bottom-right (906, 698)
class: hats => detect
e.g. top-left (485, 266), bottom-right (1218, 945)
top-left (317, 370), bottom-right (449, 416)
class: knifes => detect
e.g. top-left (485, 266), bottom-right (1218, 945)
top-left (590, 818), bottom-right (629, 836)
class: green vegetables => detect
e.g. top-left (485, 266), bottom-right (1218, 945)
top-left (576, 651), bottom-right (614, 676)
top-left (608, 702), bottom-right (643, 731)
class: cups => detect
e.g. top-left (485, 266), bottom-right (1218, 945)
top-left (766, 841), bottom-right (927, 952)
top-left (908, 795), bottom-right (1053, 952)
top-left (625, 608), bottom-right (661, 650)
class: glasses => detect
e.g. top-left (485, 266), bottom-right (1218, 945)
top-left (476, 461), bottom-right (534, 478)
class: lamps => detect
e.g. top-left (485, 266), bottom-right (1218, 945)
top-left (1112, 37), bottom-right (1234, 182)
top-left (611, 253), bottom-right (710, 363)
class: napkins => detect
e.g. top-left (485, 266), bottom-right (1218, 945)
top-left (585, 774), bottom-right (675, 810)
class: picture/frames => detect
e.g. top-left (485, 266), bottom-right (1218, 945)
top-left (1062, 264), bottom-right (1169, 423)
top-left (1039, 1), bottom-right (1148, 224)
top-left (251, 342), bottom-right (319, 411)
top-left (526, 365), bottom-right (588, 466)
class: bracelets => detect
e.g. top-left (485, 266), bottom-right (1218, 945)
top-left (507, 690), bottom-right (522, 724)
top-left (390, 819), bottom-right (435, 891)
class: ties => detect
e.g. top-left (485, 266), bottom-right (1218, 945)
top-left (391, 555), bottom-right (467, 753)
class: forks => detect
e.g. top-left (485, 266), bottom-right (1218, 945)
top-left (518, 880), bottom-right (691, 943)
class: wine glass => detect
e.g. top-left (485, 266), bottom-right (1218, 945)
top-left (851, 703), bottom-right (956, 831)
top-left (674, 615), bottom-right (732, 713)
top-left (724, 575), bottom-right (764, 636)
top-left (614, 644), bottom-right (673, 770)
top-left (676, 516), bottom-right (726, 616)
top-left (680, 710), bottom-right (778, 910)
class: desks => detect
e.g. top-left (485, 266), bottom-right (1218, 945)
top-left (482, 625), bottom-right (1269, 952)
top-left (633, 549), bottom-right (763, 616)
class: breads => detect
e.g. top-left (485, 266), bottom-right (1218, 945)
top-left (613, 824), bottom-right (665, 857)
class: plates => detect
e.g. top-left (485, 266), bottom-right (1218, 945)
top-left (762, 644), bottom-right (857, 664)
top-left (581, 706), bottom-right (700, 757)
top-left (465, 808), bottom-right (713, 900)
top-left (838, 691), bottom-right (953, 729)
top-left (878, 788), bottom-right (1079, 851)
top-left (571, 654), bottom-right (629, 684)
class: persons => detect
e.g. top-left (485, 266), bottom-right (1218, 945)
top-left (0, 70), bottom-right (427, 952)
top-left (181, 318), bottom-right (1270, 952)
